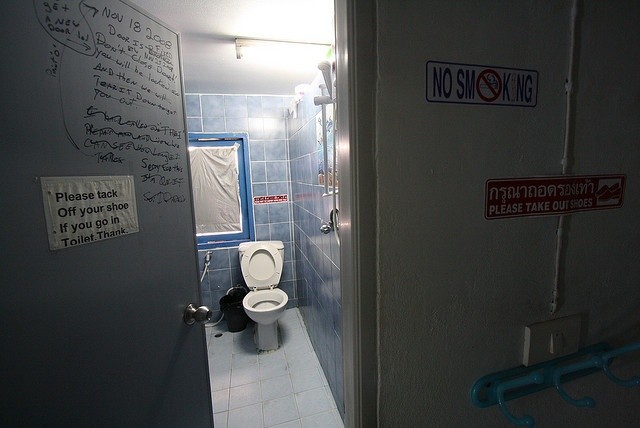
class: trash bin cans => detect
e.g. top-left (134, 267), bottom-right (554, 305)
top-left (219, 293), bottom-right (248, 332)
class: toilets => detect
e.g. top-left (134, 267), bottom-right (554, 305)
top-left (238, 240), bottom-right (288, 351)
